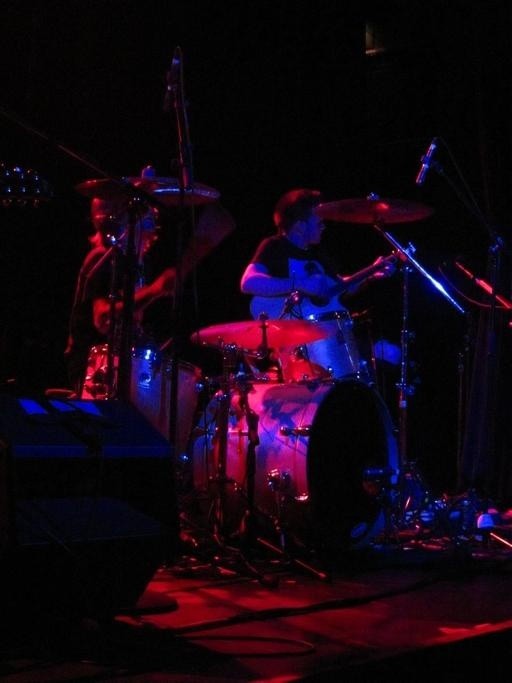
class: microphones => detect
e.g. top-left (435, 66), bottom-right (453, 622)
top-left (162, 47), bottom-right (182, 110)
top-left (416, 134), bottom-right (439, 186)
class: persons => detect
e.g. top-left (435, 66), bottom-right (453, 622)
top-left (239, 187), bottom-right (399, 296)
top-left (65, 189), bottom-right (236, 473)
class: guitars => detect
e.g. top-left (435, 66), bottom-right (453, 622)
top-left (250, 242), bottom-right (417, 321)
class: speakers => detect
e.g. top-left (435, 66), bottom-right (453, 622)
top-left (0, 400), bottom-right (179, 659)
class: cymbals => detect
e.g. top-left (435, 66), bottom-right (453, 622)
top-left (80, 176), bottom-right (218, 204)
top-left (313, 195), bottom-right (440, 224)
top-left (190, 320), bottom-right (326, 348)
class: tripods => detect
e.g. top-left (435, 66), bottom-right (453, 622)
top-left (171, 267), bottom-right (511, 582)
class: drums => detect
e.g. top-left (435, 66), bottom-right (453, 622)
top-left (81, 343), bottom-right (201, 459)
top-left (179, 373), bottom-right (399, 547)
